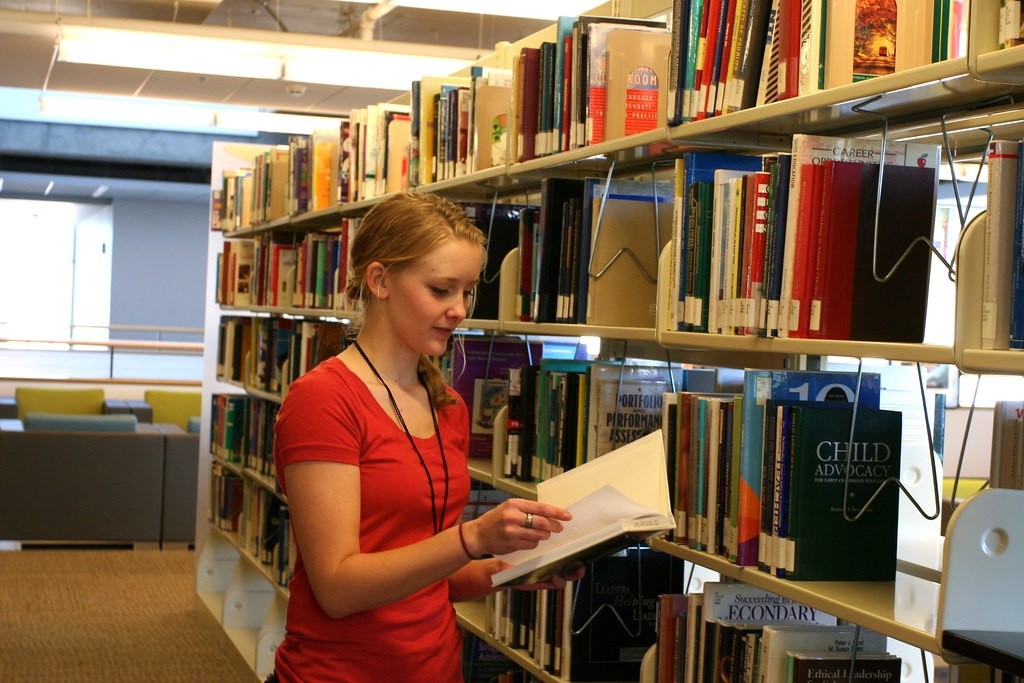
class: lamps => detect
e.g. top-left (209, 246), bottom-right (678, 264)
top-left (56, 22), bottom-right (483, 92)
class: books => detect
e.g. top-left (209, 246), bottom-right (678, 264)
top-left (208, 0), bottom-right (1024, 683)
top-left (491, 430), bottom-right (676, 590)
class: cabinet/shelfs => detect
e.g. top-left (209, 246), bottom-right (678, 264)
top-left (198, 0), bottom-right (1024, 683)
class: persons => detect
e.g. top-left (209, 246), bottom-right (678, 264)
top-left (265, 191), bottom-right (584, 683)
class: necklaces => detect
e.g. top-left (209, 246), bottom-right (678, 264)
top-left (352, 339), bottom-right (448, 536)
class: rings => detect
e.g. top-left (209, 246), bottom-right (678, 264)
top-left (527, 513), bottom-right (532, 528)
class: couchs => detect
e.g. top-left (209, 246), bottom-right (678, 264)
top-left (0, 388), bottom-right (202, 550)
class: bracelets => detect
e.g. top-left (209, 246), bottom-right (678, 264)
top-left (459, 521), bottom-right (480, 560)
